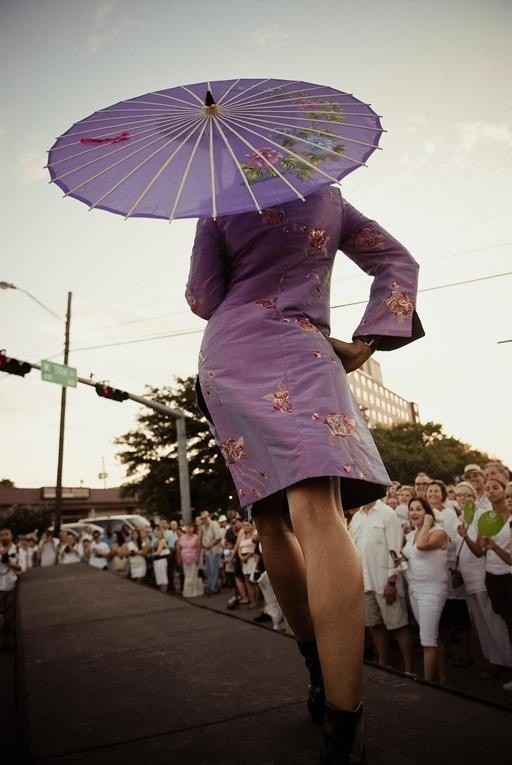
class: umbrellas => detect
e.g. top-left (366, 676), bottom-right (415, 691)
top-left (43, 78), bottom-right (387, 222)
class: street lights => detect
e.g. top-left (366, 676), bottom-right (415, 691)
top-left (0, 280), bottom-right (71, 537)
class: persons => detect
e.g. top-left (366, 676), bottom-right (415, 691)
top-left (186, 182), bottom-right (426, 765)
top-left (345, 463), bottom-right (511, 690)
top-left (179, 511), bottom-right (295, 636)
top-left (1, 527), bottom-right (82, 634)
top-left (76, 517), bottom-right (184, 592)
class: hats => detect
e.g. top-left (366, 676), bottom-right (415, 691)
top-left (218, 514), bottom-right (227, 523)
top-left (198, 510), bottom-right (212, 520)
top-left (460, 464), bottom-right (484, 480)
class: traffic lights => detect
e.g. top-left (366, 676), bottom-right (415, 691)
top-left (0, 354), bottom-right (32, 377)
top-left (96, 383), bottom-right (128, 402)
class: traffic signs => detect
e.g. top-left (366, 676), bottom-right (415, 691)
top-left (41, 359), bottom-right (77, 388)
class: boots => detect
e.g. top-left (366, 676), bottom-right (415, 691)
top-left (297, 638), bottom-right (364, 763)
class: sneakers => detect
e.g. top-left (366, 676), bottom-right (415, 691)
top-left (236, 595), bottom-right (282, 631)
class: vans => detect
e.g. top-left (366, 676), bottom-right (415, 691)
top-left (48, 514), bottom-right (153, 537)
